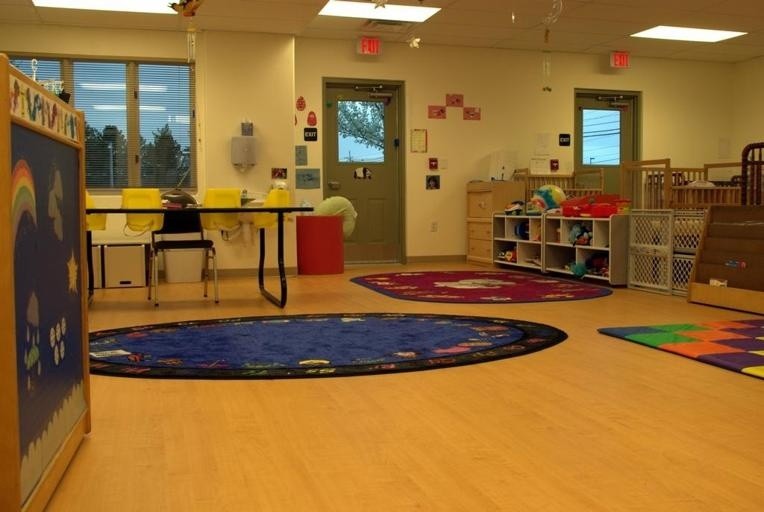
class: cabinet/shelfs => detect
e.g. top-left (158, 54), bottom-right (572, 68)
top-left (687, 204), bottom-right (764, 315)
top-left (466, 182), bottom-right (630, 286)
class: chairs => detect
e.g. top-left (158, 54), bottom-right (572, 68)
top-left (145, 190), bottom-right (220, 305)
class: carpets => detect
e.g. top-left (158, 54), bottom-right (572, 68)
top-left (88, 312), bottom-right (569, 381)
top-left (596, 318), bottom-right (764, 382)
top-left (349, 269), bottom-right (614, 303)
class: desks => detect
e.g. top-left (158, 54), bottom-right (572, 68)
top-left (85, 207), bottom-right (314, 308)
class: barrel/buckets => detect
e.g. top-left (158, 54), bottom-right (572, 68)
top-left (296, 215), bottom-right (344, 274)
